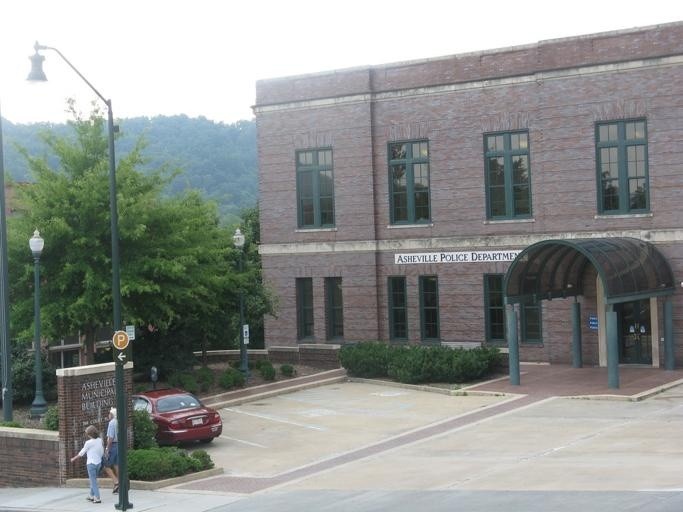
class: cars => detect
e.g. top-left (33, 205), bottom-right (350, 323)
top-left (132, 386), bottom-right (222, 445)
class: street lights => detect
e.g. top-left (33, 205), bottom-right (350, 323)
top-left (233, 228), bottom-right (248, 373)
top-left (27, 43), bottom-right (133, 512)
top-left (29, 228), bottom-right (47, 419)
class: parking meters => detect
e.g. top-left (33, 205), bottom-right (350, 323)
top-left (150, 367), bottom-right (158, 389)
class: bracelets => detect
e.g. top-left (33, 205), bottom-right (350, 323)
top-left (106, 448), bottom-right (108, 451)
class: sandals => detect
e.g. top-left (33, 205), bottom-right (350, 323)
top-left (93, 500), bottom-right (101, 503)
top-left (86, 497), bottom-right (94, 501)
top-left (112, 483), bottom-right (120, 493)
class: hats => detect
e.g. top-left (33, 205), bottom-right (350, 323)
top-left (109, 406), bottom-right (117, 419)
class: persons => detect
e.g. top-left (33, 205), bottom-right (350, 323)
top-left (103, 408), bottom-right (119, 494)
top-left (70, 425), bottom-right (105, 503)
